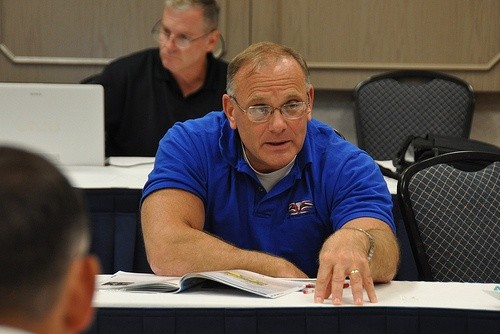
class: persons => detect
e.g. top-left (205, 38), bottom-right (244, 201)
top-left (80, 0), bottom-right (229, 157)
top-left (139, 41), bottom-right (399, 305)
top-left (0, 145), bottom-right (96, 334)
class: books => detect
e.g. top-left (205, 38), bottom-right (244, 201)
top-left (97, 269), bottom-right (306, 298)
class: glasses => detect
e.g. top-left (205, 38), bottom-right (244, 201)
top-left (151, 19), bottom-right (216, 49)
top-left (230, 92), bottom-right (310, 122)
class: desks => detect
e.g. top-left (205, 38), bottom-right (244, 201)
top-left (56, 156), bottom-right (420, 282)
top-left (83, 275), bottom-right (500, 334)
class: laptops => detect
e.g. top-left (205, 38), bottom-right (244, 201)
top-left (0, 82), bottom-right (111, 166)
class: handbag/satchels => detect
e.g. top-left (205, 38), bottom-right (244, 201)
top-left (392, 132), bottom-right (500, 176)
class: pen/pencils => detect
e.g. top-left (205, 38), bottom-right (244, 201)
top-left (304, 283), bottom-right (350, 294)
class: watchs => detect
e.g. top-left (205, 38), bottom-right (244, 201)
top-left (343, 228), bottom-right (375, 261)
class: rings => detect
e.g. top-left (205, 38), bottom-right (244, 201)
top-left (350, 269), bottom-right (359, 275)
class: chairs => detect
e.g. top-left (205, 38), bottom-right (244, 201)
top-left (351, 68), bottom-right (475, 161)
top-left (397, 150), bottom-right (500, 284)
